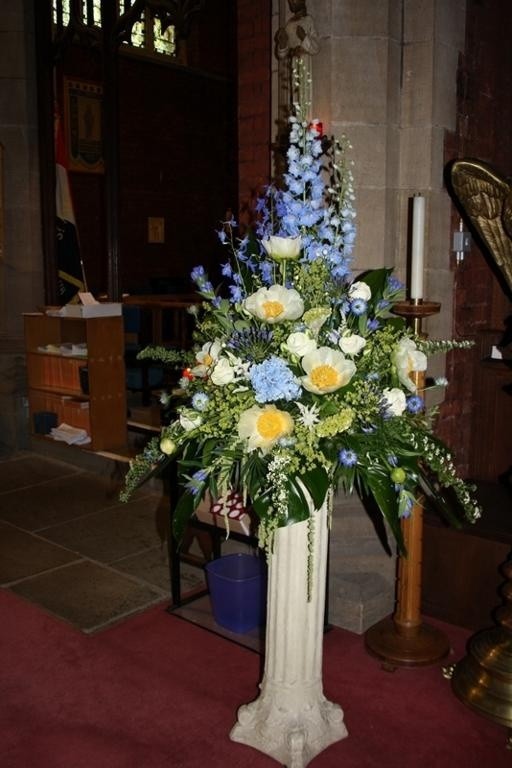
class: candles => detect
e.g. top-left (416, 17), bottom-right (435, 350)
top-left (411, 196), bottom-right (426, 301)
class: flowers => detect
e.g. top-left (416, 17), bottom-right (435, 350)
top-left (117, 105), bottom-right (489, 600)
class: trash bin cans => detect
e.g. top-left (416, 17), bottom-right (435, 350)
top-left (204, 552), bottom-right (268, 634)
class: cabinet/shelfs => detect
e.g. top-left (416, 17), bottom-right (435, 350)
top-left (21, 310), bottom-right (128, 451)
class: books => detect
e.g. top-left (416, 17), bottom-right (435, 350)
top-left (21, 290), bottom-right (123, 319)
top-left (46, 422), bottom-right (92, 445)
top-left (38, 341), bottom-right (88, 357)
